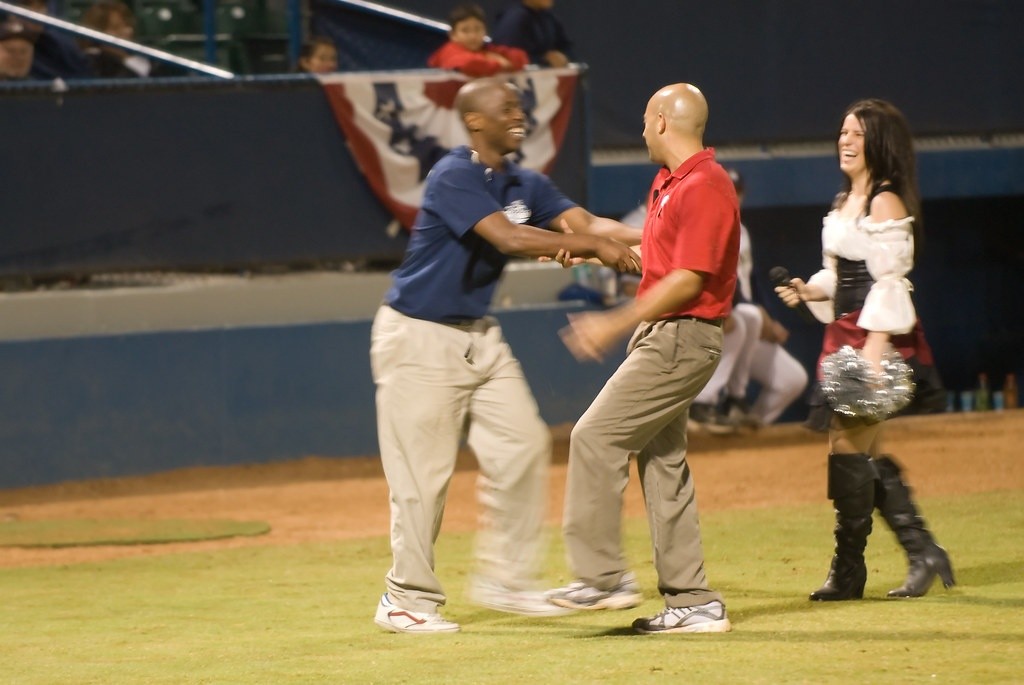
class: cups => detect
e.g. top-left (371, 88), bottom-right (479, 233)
top-left (960, 391), bottom-right (974, 411)
top-left (992, 391), bottom-right (1004, 408)
top-left (945, 391), bottom-right (955, 411)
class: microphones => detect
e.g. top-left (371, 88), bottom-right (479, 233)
top-left (769, 265), bottom-right (815, 324)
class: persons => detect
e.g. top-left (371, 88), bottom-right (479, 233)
top-left (622, 170), bottom-right (808, 434)
top-left (774, 99), bottom-right (956, 602)
top-left (539, 83), bottom-right (741, 634)
top-left (427, 7), bottom-right (527, 77)
top-left (297, 36), bottom-right (337, 73)
top-left (0, 0), bottom-right (165, 79)
top-left (491, 0), bottom-right (572, 68)
top-left (370, 77), bottom-right (643, 635)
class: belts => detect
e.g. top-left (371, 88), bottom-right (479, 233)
top-left (681, 315), bottom-right (722, 327)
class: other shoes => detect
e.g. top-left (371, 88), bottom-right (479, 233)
top-left (688, 403), bottom-right (736, 436)
top-left (725, 399), bottom-right (763, 429)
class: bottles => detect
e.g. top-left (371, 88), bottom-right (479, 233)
top-left (974, 373), bottom-right (990, 410)
top-left (1004, 373), bottom-right (1017, 407)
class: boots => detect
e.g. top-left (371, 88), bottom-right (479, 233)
top-left (864, 453), bottom-right (955, 600)
top-left (808, 453), bottom-right (878, 602)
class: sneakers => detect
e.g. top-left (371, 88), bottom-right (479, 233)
top-left (374, 593), bottom-right (461, 634)
top-left (544, 571), bottom-right (644, 611)
top-left (632, 602), bottom-right (731, 635)
top-left (466, 580), bottom-right (570, 616)
